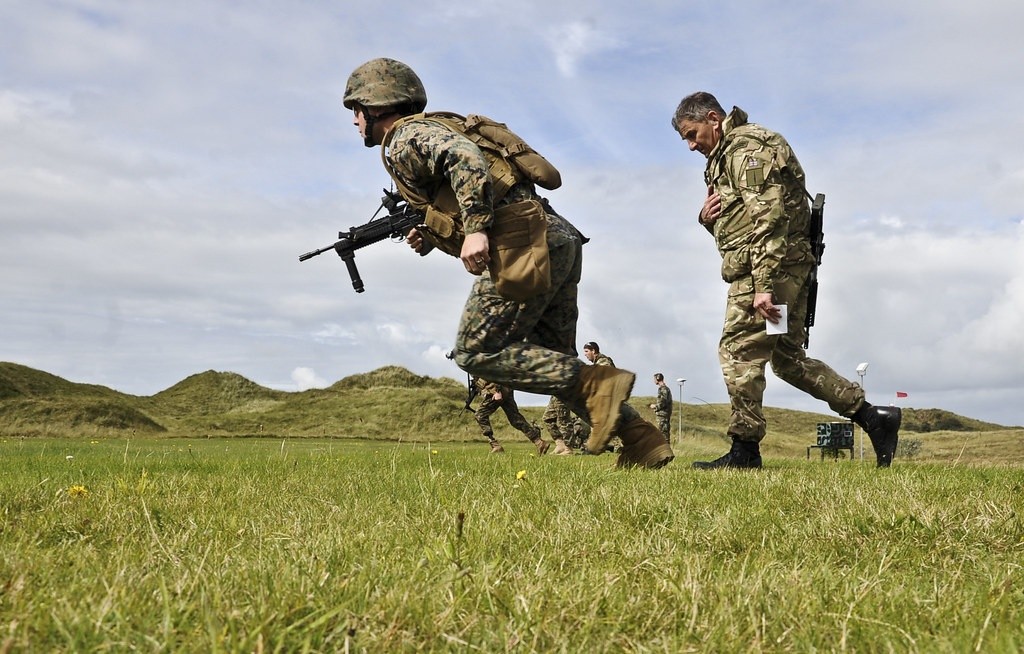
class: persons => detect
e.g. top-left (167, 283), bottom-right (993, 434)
top-left (571, 410), bottom-right (591, 452)
top-left (343, 57), bottom-right (675, 468)
top-left (650, 372), bottom-right (672, 443)
top-left (543, 396), bottom-right (574, 454)
top-left (671, 91), bottom-right (901, 469)
top-left (583, 341), bottom-right (622, 453)
top-left (446, 349), bottom-right (551, 455)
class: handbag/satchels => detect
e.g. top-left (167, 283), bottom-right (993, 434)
top-left (487, 200), bottom-right (552, 300)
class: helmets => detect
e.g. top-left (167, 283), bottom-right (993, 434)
top-left (342, 58), bottom-right (427, 113)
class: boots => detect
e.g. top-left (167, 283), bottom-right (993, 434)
top-left (489, 438), bottom-right (504, 455)
top-left (854, 402), bottom-right (901, 468)
top-left (694, 435), bottom-right (762, 470)
top-left (534, 437), bottom-right (550, 455)
top-left (615, 420), bottom-right (675, 471)
top-left (551, 441), bottom-right (571, 456)
top-left (577, 363), bottom-right (637, 454)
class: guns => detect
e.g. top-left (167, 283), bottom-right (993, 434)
top-left (458, 385), bottom-right (482, 419)
top-left (297, 184), bottom-right (426, 294)
top-left (802, 191), bottom-right (826, 350)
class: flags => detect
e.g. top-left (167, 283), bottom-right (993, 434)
top-left (897, 392), bottom-right (907, 397)
